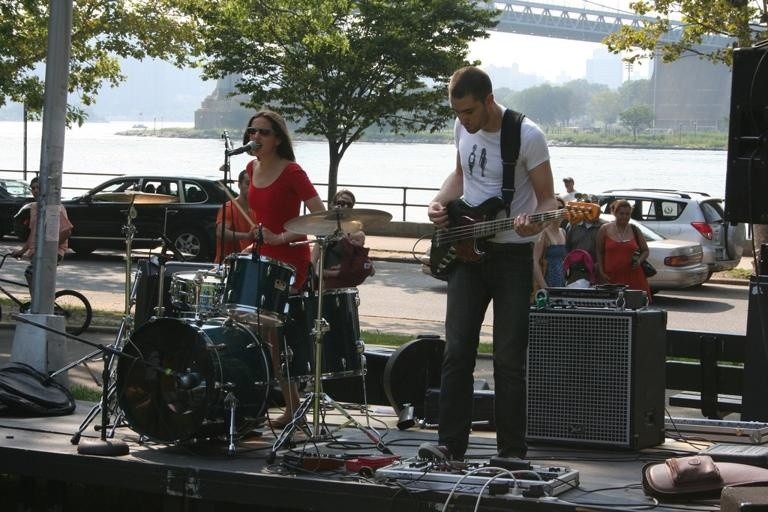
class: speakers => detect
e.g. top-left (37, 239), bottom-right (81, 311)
top-left (723, 46), bottom-right (768, 226)
top-left (524, 307), bottom-right (667, 455)
top-left (739, 273), bottom-right (768, 423)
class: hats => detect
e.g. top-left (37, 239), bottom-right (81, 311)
top-left (563, 177), bottom-right (574, 183)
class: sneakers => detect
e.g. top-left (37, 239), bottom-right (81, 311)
top-left (270, 414), bottom-right (307, 428)
top-left (418, 442), bottom-right (464, 461)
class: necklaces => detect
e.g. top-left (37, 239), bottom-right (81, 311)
top-left (611, 221), bottom-right (630, 243)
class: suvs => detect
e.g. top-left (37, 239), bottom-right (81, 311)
top-left (580, 190), bottom-right (747, 272)
top-left (14, 178), bottom-right (238, 259)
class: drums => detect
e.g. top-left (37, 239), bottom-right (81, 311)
top-left (117, 315), bottom-right (273, 442)
top-left (229, 253), bottom-right (295, 320)
top-left (170, 271), bottom-right (227, 314)
top-left (282, 288), bottom-right (365, 385)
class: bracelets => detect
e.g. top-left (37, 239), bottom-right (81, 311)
top-left (281, 232), bottom-right (285, 244)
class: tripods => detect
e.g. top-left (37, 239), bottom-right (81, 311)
top-left (265, 239), bottom-right (395, 464)
top-left (70, 225), bottom-right (149, 446)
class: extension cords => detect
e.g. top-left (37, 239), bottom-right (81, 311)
top-left (495, 493), bottom-right (558, 503)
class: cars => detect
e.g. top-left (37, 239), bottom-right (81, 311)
top-left (597, 214), bottom-right (707, 289)
top-left (0, 179), bottom-right (31, 242)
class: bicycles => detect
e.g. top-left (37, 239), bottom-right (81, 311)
top-left (1, 248), bottom-right (91, 337)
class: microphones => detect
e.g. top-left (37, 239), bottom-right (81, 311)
top-left (227, 140), bottom-right (257, 156)
top-left (161, 234), bottom-right (186, 262)
top-left (178, 372), bottom-right (201, 390)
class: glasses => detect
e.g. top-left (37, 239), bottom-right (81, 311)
top-left (246, 127), bottom-right (277, 137)
top-left (335, 200), bottom-right (353, 208)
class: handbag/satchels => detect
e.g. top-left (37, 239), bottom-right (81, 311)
top-left (530, 258), bottom-right (549, 304)
top-left (641, 260), bottom-right (657, 277)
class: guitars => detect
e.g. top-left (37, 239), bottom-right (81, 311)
top-left (430, 196), bottom-right (600, 278)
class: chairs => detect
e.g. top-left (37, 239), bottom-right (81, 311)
top-left (188, 186), bottom-right (205, 201)
top-left (146, 185), bottom-right (167, 195)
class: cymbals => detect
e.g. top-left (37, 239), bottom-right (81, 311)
top-left (284, 208), bottom-right (392, 236)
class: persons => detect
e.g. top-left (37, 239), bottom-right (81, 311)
top-left (566, 191), bottom-right (610, 287)
top-left (532, 195), bottom-right (568, 292)
top-left (11, 172), bottom-right (73, 309)
top-left (304, 186), bottom-right (369, 379)
top-left (216, 171), bottom-right (259, 261)
top-left (592, 199), bottom-right (656, 312)
top-left (237, 109), bottom-right (326, 430)
top-left (558, 176), bottom-right (582, 204)
top-left (418, 60), bottom-right (556, 461)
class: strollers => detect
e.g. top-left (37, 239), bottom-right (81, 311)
top-left (561, 249), bottom-right (596, 288)
